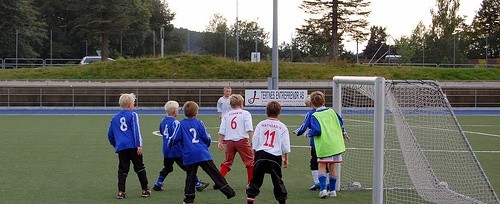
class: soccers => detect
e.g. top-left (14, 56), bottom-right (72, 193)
top-left (304, 95), bottom-right (311, 107)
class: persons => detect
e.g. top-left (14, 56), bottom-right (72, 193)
top-left (213, 94), bottom-right (254, 190)
top-left (246, 100), bottom-right (291, 204)
top-left (217, 86), bottom-right (233, 123)
top-left (293, 95), bottom-right (330, 193)
top-left (169, 100), bottom-right (236, 204)
top-left (108, 93), bottom-right (151, 199)
top-left (304, 91), bottom-right (351, 199)
top-left (153, 100), bottom-right (210, 192)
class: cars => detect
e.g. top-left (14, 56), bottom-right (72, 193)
top-left (385, 55), bottom-right (411, 63)
top-left (79, 56), bottom-right (115, 66)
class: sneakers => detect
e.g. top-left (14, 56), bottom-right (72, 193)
top-left (309, 185), bottom-right (320, 190)
top-left (141, 189), bottom-right (150, 197)
top-left (183, 198), bottom-right (194, 204)
top-left (227, 191), bottom-right (236, 199)
top-left (117, 191), bottom-right (128, 199)
top-left (319, 189), bottom-right (329, 198)
top-left (213, 184), bottom-right (219, 190)
top-left (327, 189), bottom-right (337, 198)
top-left (196, 180), bottom-right (209, 192)
top-left (153, 184), bottom-right (163, 191)
